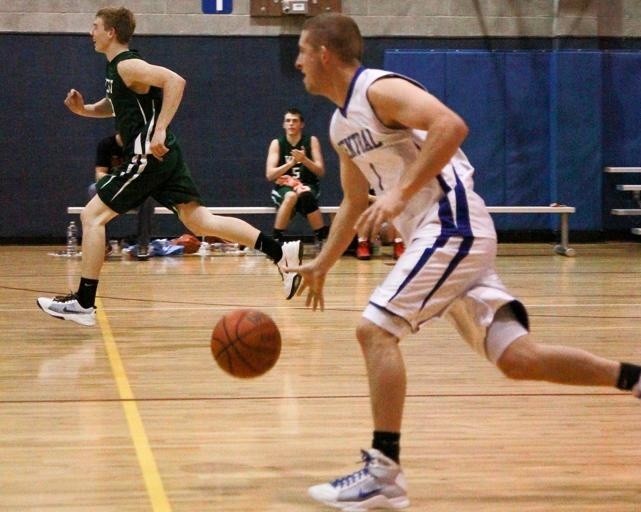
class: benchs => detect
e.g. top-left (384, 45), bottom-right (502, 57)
top-left (61, 164), bottom-right (641, 258)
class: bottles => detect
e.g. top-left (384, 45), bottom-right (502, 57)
top-left (372, 235), bottom-right (382, 256)
top-left (66, 221), bottom-right (78, 256)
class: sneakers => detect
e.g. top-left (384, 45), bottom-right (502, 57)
top-left (308, 449), bottom-right (409, 512)
top-left (276, 240), bottom-right (303, 299)
top-left (394, 242), bottom-right (404, 259)
top-left (37, 297), bottom-right (96, 327)
top-left (356, 241), bottom-right (370, 259)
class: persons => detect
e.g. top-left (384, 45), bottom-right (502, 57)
top-left (36, 6), bottom-right (306, 327)
top-left (95, 132), bottom-right (156, 258)
top-left (280, 12), bottom-right (639, 511)
top-left (265, 107), bottom-right (331, 249)
top-left (357, 189), bottom-right (407, 260)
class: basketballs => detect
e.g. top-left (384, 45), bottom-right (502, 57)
top-left (210, 308), bottom-right (282, 379)
